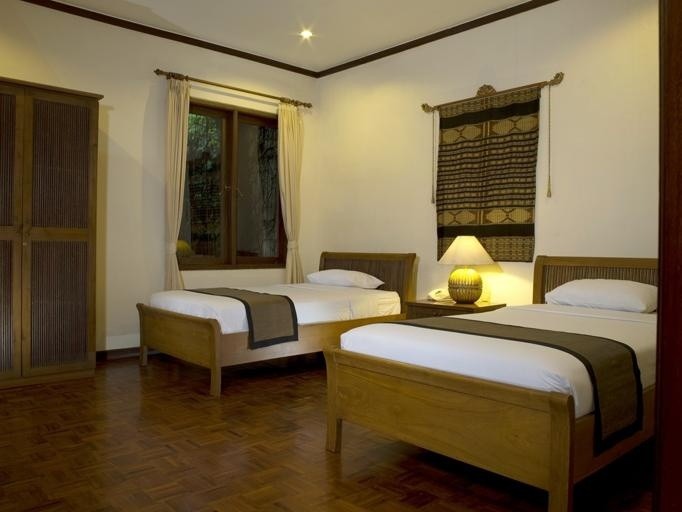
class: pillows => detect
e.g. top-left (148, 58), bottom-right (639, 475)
top-left (543, 279), bottom-right (662, 314)
top-left (302, 269), bottom-right (388, 291)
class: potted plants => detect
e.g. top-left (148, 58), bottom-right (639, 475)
top-left (170, 239), bottom-right (193, 272)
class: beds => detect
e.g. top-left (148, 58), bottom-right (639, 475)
top-left (321, 255), bottom-right (661, 508)
top-left (135, 249), bottom-right (420, 400)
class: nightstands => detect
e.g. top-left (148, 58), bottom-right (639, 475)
top-left (404, 292), bottom-right (508, 315)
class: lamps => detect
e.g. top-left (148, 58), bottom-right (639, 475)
top-left (436, 232), bottom-right (502, 304)
